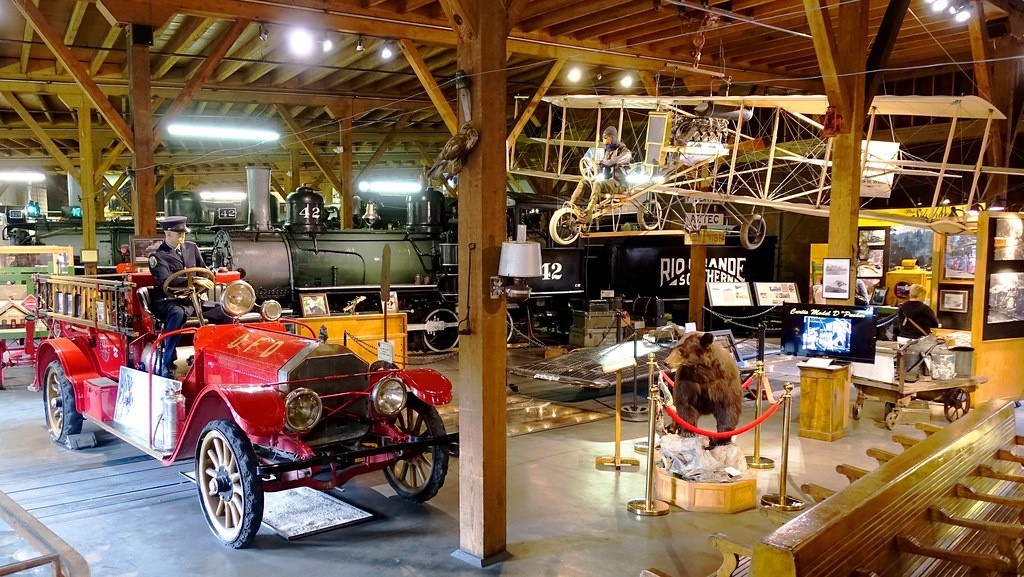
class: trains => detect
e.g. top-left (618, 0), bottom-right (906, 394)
top-left (990, 285), bottom-right (1024, 318)
top-left (112, 164), bottom-right (780, 353)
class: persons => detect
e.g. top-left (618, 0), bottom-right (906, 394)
top-left (812, 278), bottom-right (826, 305)
top-left (855, 272), bottom-right (870, 305)
top-left (146, 215), bottom-right (235, 379)
top-left (897, 284), bottom-right (939, 347)
top-left (566, 123), bottom-right (631, 222)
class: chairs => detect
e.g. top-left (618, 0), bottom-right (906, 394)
top-left (137, 285), bottom-right (209, 339)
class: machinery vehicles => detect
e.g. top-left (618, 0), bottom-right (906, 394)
top-left (26, 270), bottom-right (454, 549)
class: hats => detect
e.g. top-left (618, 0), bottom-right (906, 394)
top-left (159, 215), bottom-right (191, 232)
top-left (603, 126), bottom-right (617, 138)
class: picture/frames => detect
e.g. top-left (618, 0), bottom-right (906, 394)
top-left (129, 235), bottom-right (165, 265)
top-left (822, 257), bottom-right (850, 300)
top-left (940, 289), bottom-right (968, 313)
top-left (869, 286), bottom-right (889, 306)
top-left (937, 227), bottom-right (977, 284)
top-left (380, 291), bottom-right (399, 314)
top-left (299, 293), bottom-right (331, 318)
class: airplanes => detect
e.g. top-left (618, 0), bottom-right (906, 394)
top-left (508, 90), bottom-right (1005, 251)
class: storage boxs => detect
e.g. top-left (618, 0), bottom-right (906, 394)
top-left (568, 310), bottom-right (624, 346)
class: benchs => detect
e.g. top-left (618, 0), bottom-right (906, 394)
top-left (639, 397), bottom-right (1024, 577)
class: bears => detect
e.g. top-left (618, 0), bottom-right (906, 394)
top-left (663, 330), bottom-right (742, 447)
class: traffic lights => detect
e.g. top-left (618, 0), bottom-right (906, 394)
top-left (25, 202), bottom-right (40, 215)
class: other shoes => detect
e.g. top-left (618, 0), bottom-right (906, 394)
top-left (162, 364), bottom-right (175, 379)
top-left (578, 209), bottom-right (592, 223)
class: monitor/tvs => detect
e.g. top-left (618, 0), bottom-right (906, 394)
top-left (779, 301), bottom-right (880, 367)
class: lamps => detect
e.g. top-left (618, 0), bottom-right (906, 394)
top-left (381, 40), bottom-right (391, 55)
top-left (356, 38), bottom-right (366, 51)
top-left (259, 24), bottom-right (270, 41)
top-left (489, 239), bottom-right (543, 301)
top-left (333, 146), bottom-right (344, 153)
top-left (924, 0), bottom-right (973, 23)
top-left (986, 16), bottom-right (1012, 39)
top-left (320, 33), bottom-right (333, 49)
top-left (594, 72), bottom-right (602, 80)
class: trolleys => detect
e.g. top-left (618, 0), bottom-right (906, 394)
top-left (852, 347), bottom-right (989, 429)
